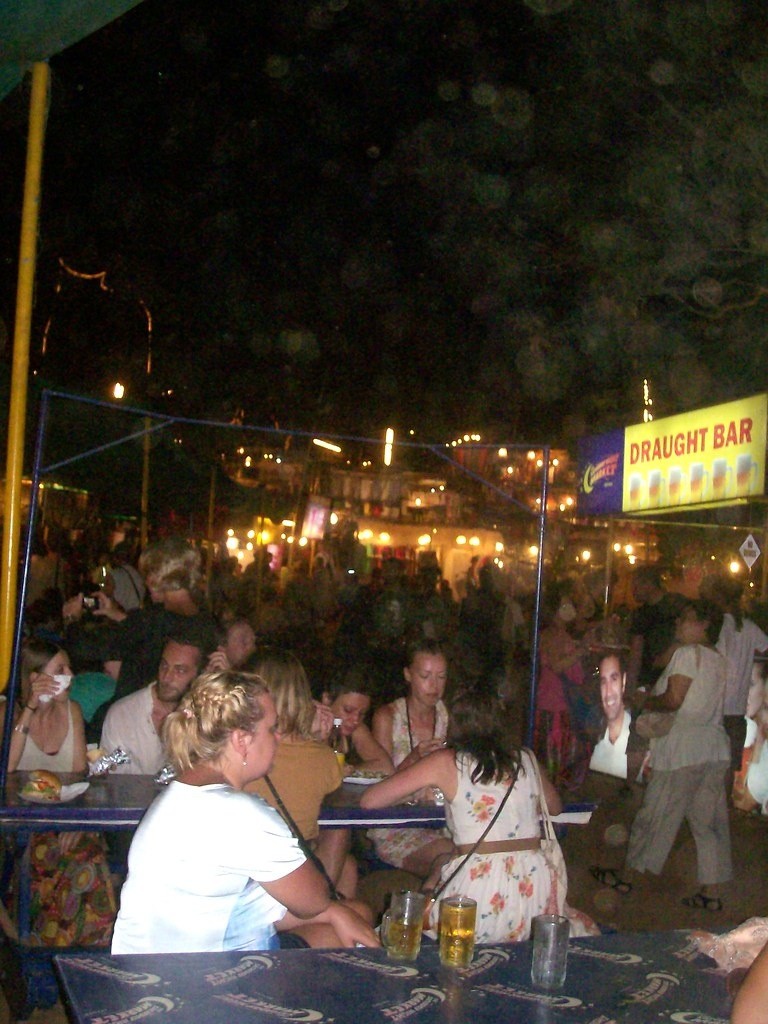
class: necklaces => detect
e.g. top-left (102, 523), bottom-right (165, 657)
top-left (406, 700), bottom-right (436, 751)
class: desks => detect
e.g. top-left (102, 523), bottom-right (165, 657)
top-left (52, 924), bottom-right (758, 1024)
top-left (0, 771), bottom-right (594, 996)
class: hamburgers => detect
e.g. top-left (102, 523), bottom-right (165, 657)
top-left (24, 769), bottom-right (62, 803)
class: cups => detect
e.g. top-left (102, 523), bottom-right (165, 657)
top-left (437, 897), bottom-right (477, 971)
top-left (380, 889), bottom-right (426, 962)
top-left (531, 914), bottom-right (571, 990)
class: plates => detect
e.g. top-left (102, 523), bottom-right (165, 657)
top-left (16, 782), bottom-right (90, 804)
top-left (343, 778), bottom-right (383, 785)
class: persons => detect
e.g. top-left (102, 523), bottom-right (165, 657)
top-left (586, 650), bottom-right (633, 778)
top-left (733, 661), bottom-right (768, 814)
top-left (0, 507), bottom-right (768, 1008)
top-left (731, 941), bottom-right (768, 1024)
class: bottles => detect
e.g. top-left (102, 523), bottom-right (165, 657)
top-left (327, 718), bottom-right (346, 773)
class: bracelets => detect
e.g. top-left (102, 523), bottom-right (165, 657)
top-left (26, 704), bottom-right (36, 711)
top-left (13, 723), bottom-right (29, 734)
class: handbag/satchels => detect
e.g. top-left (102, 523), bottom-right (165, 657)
top-left (635, 647), bottom-right (700, 738)
top-left (539, 840), bottom-right (569, 923)
top-left (422, 894), bottom-right (435, 930)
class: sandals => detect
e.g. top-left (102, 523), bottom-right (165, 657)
top-left (590, 864), bottom-right (632, 895)
top-left (681, 893), bottom-right (722, 913)
